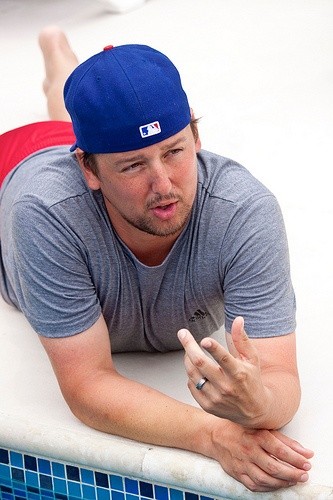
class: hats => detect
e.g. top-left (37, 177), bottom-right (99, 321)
top-left (63, 44), bottom-right (191, 154)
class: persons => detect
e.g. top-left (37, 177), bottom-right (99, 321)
top-left (0, 25), bottom-right (314, 493)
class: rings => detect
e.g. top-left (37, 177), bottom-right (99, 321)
top-left (195, 377), bottom-right (208, 390)
